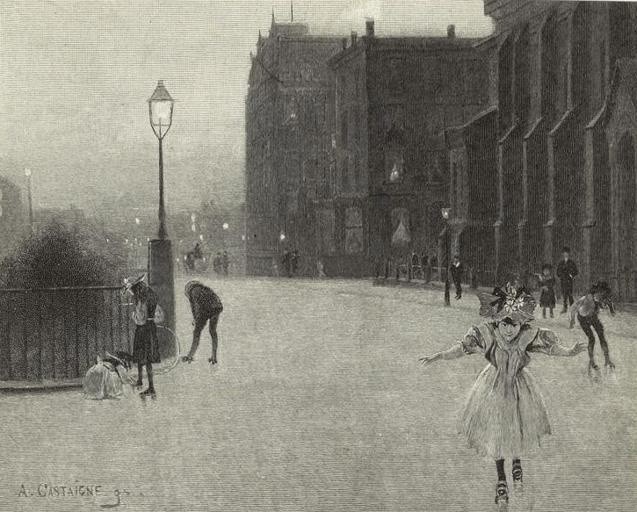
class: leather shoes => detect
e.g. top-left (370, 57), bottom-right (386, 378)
top-left (543, 308), bottom-right (567, 319)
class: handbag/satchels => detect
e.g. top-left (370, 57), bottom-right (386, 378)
top-left (131, 303), bottom-right (164, 326)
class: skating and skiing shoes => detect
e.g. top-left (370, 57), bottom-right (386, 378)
top-left (588, 361), bottom-right (600, 372)
top-left (180, 356), bottom-right (193, 364)
top-left (130, 380), bottom-right (141, 392)
top-left (604, 360), bottom-right (615, 370)
top-left (495, 480), bottom-right (508, 509)
top-left (208, 358), bottom-right (216, 364)
top-left (139, 388), bottom-right (156, 403)
top-left (511, 464), bottom-right (523, 490)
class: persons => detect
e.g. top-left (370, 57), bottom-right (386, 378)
top-left (537, 261), bottom-right (557, 320)
top-left (370, 250), bottom-right (434, 281)
top-left (177, 243), bottom-right (233, 280)
top-left (124, 280), bottom-right (162, 401)
top-left (565, 277), bottom-right (619, 373)
top-left (176, 278), bottom-right (223, 366)
top-left (448, 254), bottom-right (464, 300)
top-left (414, 281), bottom-right (587, 504)
top-left (316, 258), bottom-right (326, 277)
top-left (555, 246), bottom-right (579, 315)
top-left (80, 350), bottom-right (137, 400)
top-left (277, 245), bottom-right (301, 278)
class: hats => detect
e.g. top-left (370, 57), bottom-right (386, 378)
top-left (184, 278), bottom-right (199, 299)
top-left (119, 275), bottom-right (145, 295)
